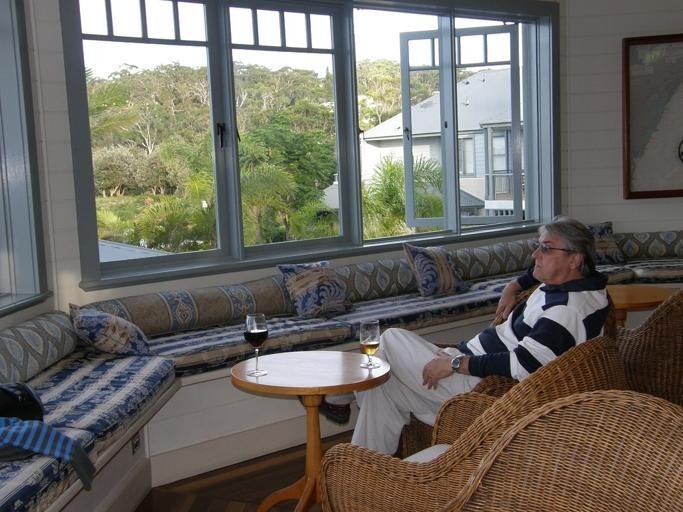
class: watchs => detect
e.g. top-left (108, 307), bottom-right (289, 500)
top-left (451, 355), bottom-right (466, 373)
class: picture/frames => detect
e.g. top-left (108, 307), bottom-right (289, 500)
top-left (621, 33), bottom-right (683, 199)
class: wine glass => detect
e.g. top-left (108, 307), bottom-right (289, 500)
top-left (244, 314), bottom-right (269, 378)
top-left (359, 319), bottom-right (381, 369)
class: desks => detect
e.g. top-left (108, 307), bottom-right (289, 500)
top-left (605, 284), bottom-right (683, 334)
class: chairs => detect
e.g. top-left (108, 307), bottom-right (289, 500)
top-left (314, 336), bottom-right (634, 512)
top-left (399, 282), bottom-right (618, 460)
top-left (433, 390), bottom-right (683, 512)
top-left (616, 288), bottom-right (683, 408)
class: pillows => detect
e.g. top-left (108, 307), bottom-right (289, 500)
top-left (402, 243), bottom-right (467, 301)
top-left (332, 258), bottom-right (420, 304)
top-left (0, 310), bottom-right (80, 385)
top-left (585, 221), bottom-right (626, 266)
top-left (613, 230), bottom-right (683, 261)
top-left (81, 273), bottom-right (298, 340)
top-left (448, 237), bottom-right (540, 281)
top-left (277, 260), bottom-right (359, 320)
top-left (68, 303), bottom-right (152, 357)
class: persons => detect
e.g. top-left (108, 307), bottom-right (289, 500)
top-left (298, 215), bottom-right (609, 457)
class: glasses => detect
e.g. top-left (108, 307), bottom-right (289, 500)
top-left (533, 242), bottom-right (574, 255)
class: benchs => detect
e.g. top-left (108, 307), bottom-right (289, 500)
top-left (623, 259), bottom-right (683, 332)
top-left (146, 265), bottom-right (505, 489)
top-left (0, 348), bottom-right (182, 512)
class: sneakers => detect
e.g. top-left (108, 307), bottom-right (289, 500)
top-left (298, 392), bottom-right (351, 424)
